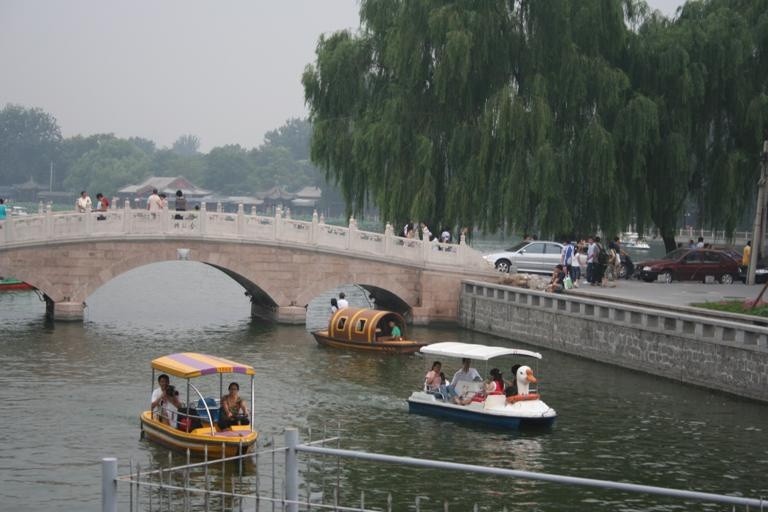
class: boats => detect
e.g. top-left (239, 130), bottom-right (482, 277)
top-left (149, 450), bottom-right (259, 512)
top-left (406, 340), bottom-right (559, 431)
top-left (4, 206), bottom-right (29, 218)
top-left (634, 245), bottom-right (742, 286)
top-left (137, 350), bottom-right (261, 465)
top-left (480, 238), bottom-right (592, 278)
top-left (706, 243), bottom-right (742, 264)
top-left (308, 304), bottom-right (428, 356)
top-left (619, 231), bottom-right (651, 249)
top-left (736, 256), bottom-right (767, 285)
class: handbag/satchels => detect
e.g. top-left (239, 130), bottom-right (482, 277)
top-left (563, 274), bottom-right (573, 290)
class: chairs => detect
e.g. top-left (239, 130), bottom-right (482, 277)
top-left (474, 393), bottom-right (508, 406)
top-left (196, 395), bottom-right (222, 423)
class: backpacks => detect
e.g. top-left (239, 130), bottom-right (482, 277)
top-left (596, 243), bottom-right (609, 264)
top-left (609, 248), bottom-right (616, 263)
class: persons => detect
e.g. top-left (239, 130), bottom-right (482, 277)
top-left (531, 234), bottom-right (537, 240)
top-left (218, 383), bottom-right (249, 430)
top-left (77, 190), bottom-right (93, 210)
top-left (382, 319), bottom-right (401, 340)
top-left (91, 194), bottom-right (109, 212)
top-left (152, 375), bottom-right (182, 428)
top-left (677, 237), bottom-right (714, 250)
top-left (147, 188), bottom-right (186, 220)
top-left (524, 233), bottom-right (531, 240)
top-left (368, 294), bottom-right (375, 309)
top-left (403, 219), bottom-right (451, 250)
top-left (549, 236), bottom-right (630, 293)
top-left (0, 199), bottom-right (7, 219)
top-left (338, 292), bottom-right (349, 309)
top-left (330, 298), bottom-right (340, 318)
top-left (743, 241), bottom-right (750, 285)
top-left (425, 358), bottom-right (522, 406)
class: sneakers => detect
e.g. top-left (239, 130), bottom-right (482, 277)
top-left (573, 281), bottom-right (619, 288)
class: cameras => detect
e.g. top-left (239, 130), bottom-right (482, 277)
top-left (167, 386), bottom-right (179, 396)
